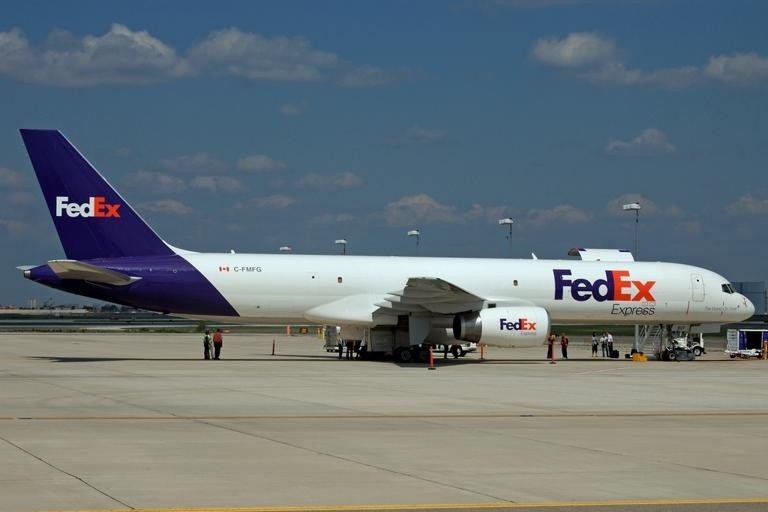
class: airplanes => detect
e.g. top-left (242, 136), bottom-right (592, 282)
top-left (16, 129), bottom-right (756, 362)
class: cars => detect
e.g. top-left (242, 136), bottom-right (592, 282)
top-left (432, 342), bottom-right (476, 356)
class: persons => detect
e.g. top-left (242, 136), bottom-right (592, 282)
top-left (591, 331), bottom-right (598, 357)
top-left (203, 329), bottom-right (214, 359)
top-left (204, 331), bottom-right (210, 359)
top-left (213, 328), bottom-right (222, 360)
top-left (336, 333), bottom-right (361, 360)
top-left (547, 328), bottom-right (568, 359)
top-left (606, 330), bottom-right (613, 357)
top-left (599, 331), bottom-right (608, 357)
top-left (693, 334), bottom-right (700, 343)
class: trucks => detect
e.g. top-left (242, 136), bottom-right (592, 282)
top-left (724, 328), bottom-right (768, 358)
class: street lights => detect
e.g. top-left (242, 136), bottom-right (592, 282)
top-left (408, 228), bottom-right (421, 256)
top-left (623, 202), bottom-right (641, 261)
top-left (335, 239), bottom-right (347, 254)
top-left (498, 218), bottom-right (515, 258)
